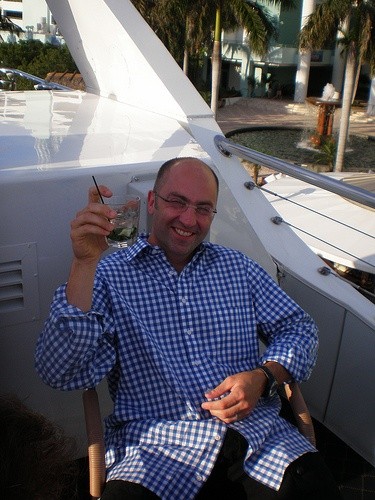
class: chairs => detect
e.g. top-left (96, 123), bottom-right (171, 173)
top-left (80, 332), bottom-right (318, 496)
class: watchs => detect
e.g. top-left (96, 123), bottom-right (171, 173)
top-left (256, 364), bottom-right (279, 399)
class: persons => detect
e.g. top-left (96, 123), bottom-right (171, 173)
top-left (35, 157), bottom-right (343, 500)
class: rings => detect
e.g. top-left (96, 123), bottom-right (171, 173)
top-left (235, 414), bottom-right (238, 421)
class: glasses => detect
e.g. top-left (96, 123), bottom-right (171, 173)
top-left (154, 190), bottom-right (217, 218)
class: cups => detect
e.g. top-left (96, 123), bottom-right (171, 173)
top-left (98, 193), bottom-right (141, 247)
